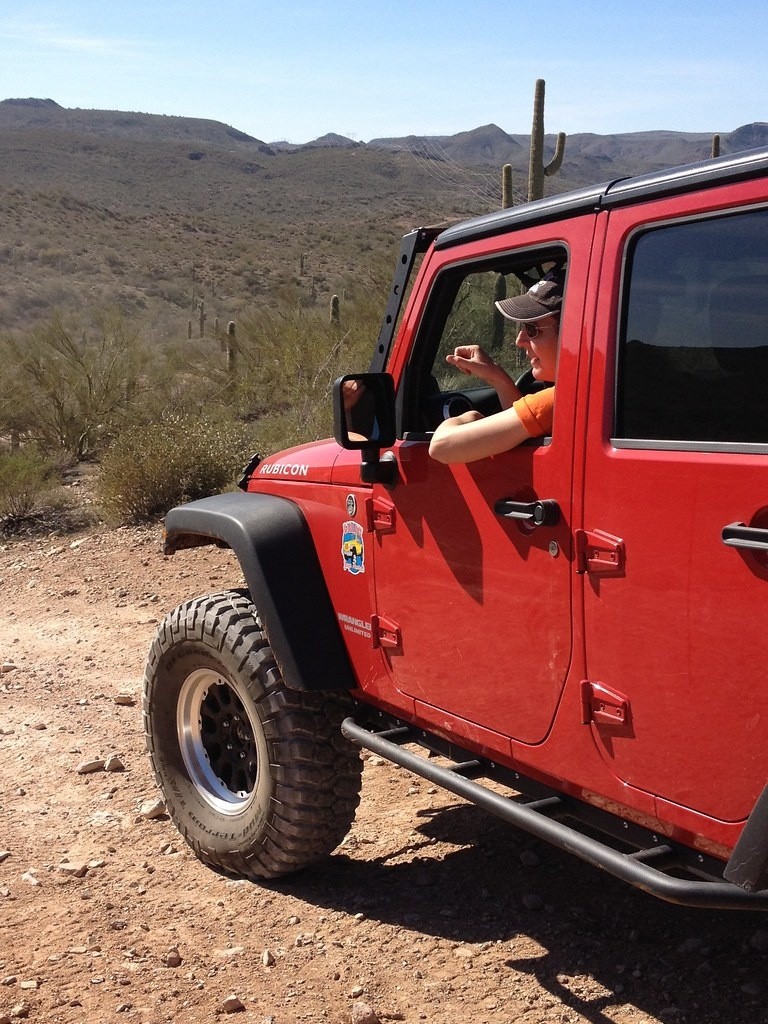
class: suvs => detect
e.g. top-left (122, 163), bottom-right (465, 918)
top-left (141, 146), bottom-right (768, 912)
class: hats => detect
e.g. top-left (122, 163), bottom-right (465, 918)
top-left (495, 268), bottom-right (566, 321)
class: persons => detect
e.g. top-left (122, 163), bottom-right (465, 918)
top-left (343, 380), bottom-right (370, 441)
top-left (428, 268), bottom-right (571, 464)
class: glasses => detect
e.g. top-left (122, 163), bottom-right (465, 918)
top-left (523, 323), bottom-right (559, 338)
